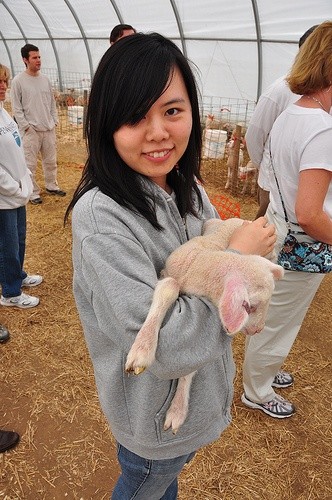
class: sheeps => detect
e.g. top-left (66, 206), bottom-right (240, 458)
top-left (224, 140), bottom-right (245, 189)
top-left (238, 160), bottom-right (258, 195)
top-left (56, 87), bottom-right (74, 109)
top-left (124, 217), bottom-right (285, 436)
top-left (205, 115), bottom-right (244, 143)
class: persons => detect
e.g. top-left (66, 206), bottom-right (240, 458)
top-left (0, 61), bottom-right (44, 309)
top-left (63, 32), bottom-right (280, 500)
top-left (239, 21), bottom-right (331, 420)
top-left (10, 43), bottom-right (68, 203)
top-left (109, 24), bottom-right (136, 46)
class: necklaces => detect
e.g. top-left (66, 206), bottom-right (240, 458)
top-left (305, 93), bottom-right (329, 114)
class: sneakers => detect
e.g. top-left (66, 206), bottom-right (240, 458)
top-left (30, 197), bottom-right (43, 205)
top-left (46, 188), bottom-right (67, 196)
top-left (1, 292), bottom-right (40, 309)
top-left (20, 274), bottom-right (44, 287)
top-left (270, 371), bottom-right (294, 388)
top-left (240, 390), bottom-right (296, 418)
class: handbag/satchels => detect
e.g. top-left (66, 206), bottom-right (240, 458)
top-left (277, 230), bottom-right (332, 274)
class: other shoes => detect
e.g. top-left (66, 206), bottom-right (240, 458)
top-left (1, 326), bottom-right (10, 342)
top-left (0, 430), bottom-right (20, 453)
